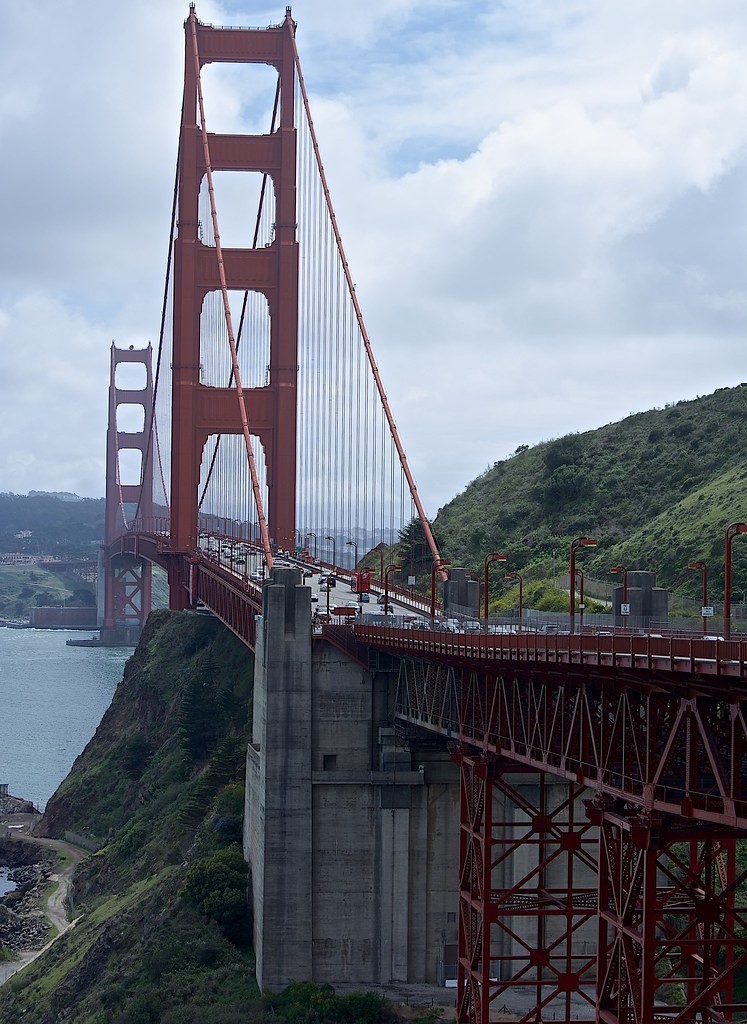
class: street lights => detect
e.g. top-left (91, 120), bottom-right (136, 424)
top-left (371, 547), bottom-right (384, 589)
top-left (430, 558), bottom-right (452, 629)
top-left (359, 567), bottom-right (376, 615)
top-left (325, 536), bottom-right (335, 572)
top-left (724, 521), bottom-right (747, 640)
top-left (610, 566), bottom-right (627, 633)
top-left (326, 569), bottom-right (345, 623)
top-left (383, 564), bottom-right (401, 614)
top-left (346, 541), bottom-right (358, 572)
top-left (568, 568), bottom-right (584, 625)
top-left (504, 572), bottom-right (523, 632)
top-left (687, 562), bottom-right (708, 636)
top-left (570, 536), bottom-right (597, 633)
top-left (307, 532), bottom-right (317, 559)
top-left (484, 552), bottom-right (508, 633)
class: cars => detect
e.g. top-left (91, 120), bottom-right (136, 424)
top-left (153, 527), bottom-right (723, 641)
